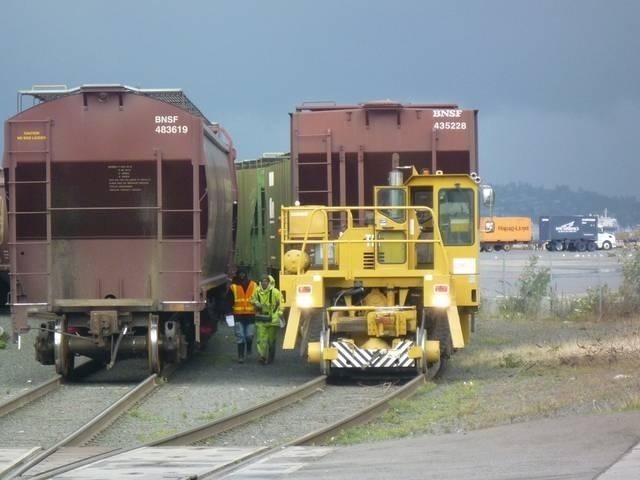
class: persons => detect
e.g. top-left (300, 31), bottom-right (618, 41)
top-left (249, 275), bottom-right (285, 364)
top-left (224, 269), bottom-right (258, 363)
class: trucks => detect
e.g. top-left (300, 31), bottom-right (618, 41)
top-left (539, 216), bottom-right (617, 250)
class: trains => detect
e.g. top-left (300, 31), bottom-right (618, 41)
top-left (235, 100), bottom-right (495, 386)
top-left (0, 78), bottom-right (242, 387)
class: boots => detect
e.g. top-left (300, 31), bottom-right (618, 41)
top-left (238, 343), bottom-right (244, 363)
top-left (247, 342), bottom-right (252, 360)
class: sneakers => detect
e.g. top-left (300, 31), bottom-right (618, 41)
top-left (258, 357), bottom-right (266, 364)
